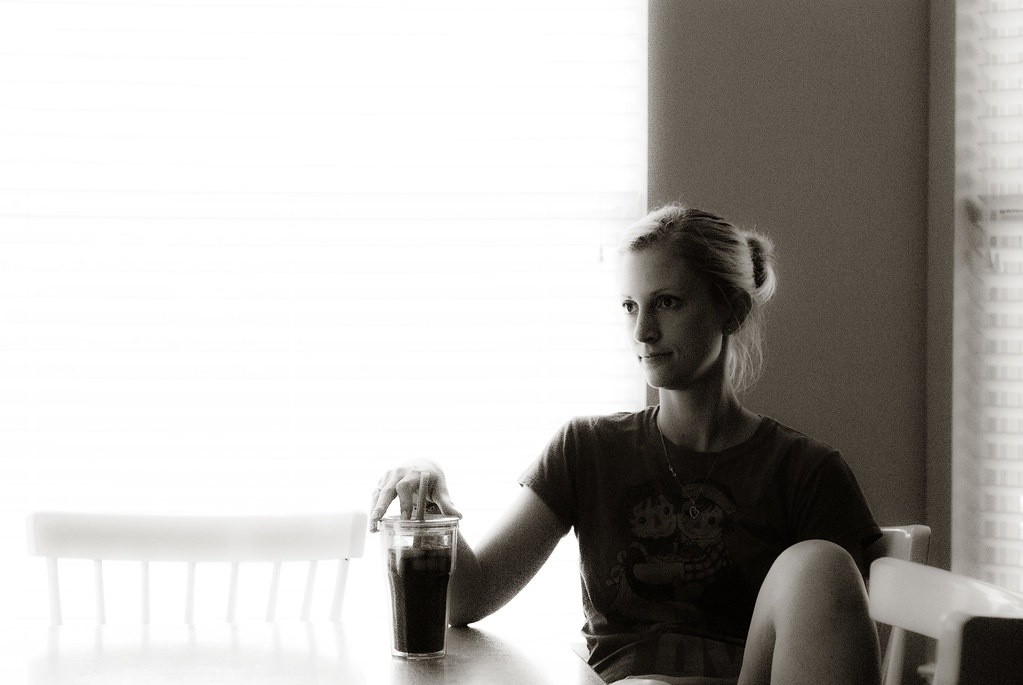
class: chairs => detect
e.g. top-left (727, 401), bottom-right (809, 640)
top-left (868, 556), bottom-right (1023, 685)
top-left (29, 499), bottom-right (368, 634)
top-left (873, 519), bottom-right (934, 673)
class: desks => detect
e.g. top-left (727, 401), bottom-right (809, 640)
top-left (1, 602), bottom-right (607, 682)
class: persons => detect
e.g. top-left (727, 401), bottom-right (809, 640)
top-left (369, 203), bottom-right (888, 685)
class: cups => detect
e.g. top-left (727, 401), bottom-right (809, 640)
top-left (380, 511), bottom-right (460, 663)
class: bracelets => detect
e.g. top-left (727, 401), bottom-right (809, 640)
top-left (413, 506), bottom-right (436, 512)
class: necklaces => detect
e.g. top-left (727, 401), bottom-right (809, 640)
top-left (656, 405), bottom-right (742, 520)
